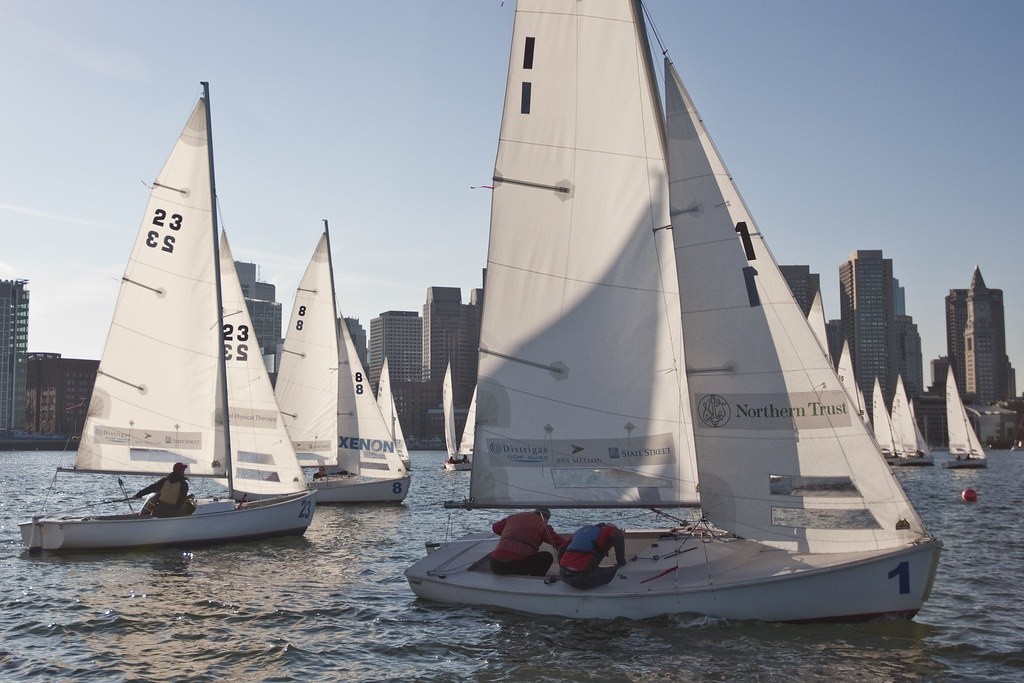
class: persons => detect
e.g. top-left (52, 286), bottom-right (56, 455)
top-left (490, 508), bottom-right (565, 577)
top-left (312, 466), bottom-right (327, 482)
top-left (449, 456), bottom-right (454, 461)
top-left (132, 461), bottom-right (195, 517)
top-left (462, 455), bottom-right (469, 463)
top-left (556, 523), bottom-right (626, 590)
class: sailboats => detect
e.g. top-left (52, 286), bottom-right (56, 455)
top-left (443, 361), bottom-right (477, 471)
top-left (807, 287), bottom-right (935, 467)
top-left (234, 219), bottom-right (412, 505)
top-left (376, 357), bottom-right (411, 472)
top-left (404, 0), bottom-right (943, 624)
top-left (17, 81), bottom-right (319, 552)
top-left (942, 365), bottom-right (988, 469)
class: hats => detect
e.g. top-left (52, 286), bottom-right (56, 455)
top-left (535, 507), bottom-right (550, 516)
top-left (173, 462), bottom-right (188, 471)
